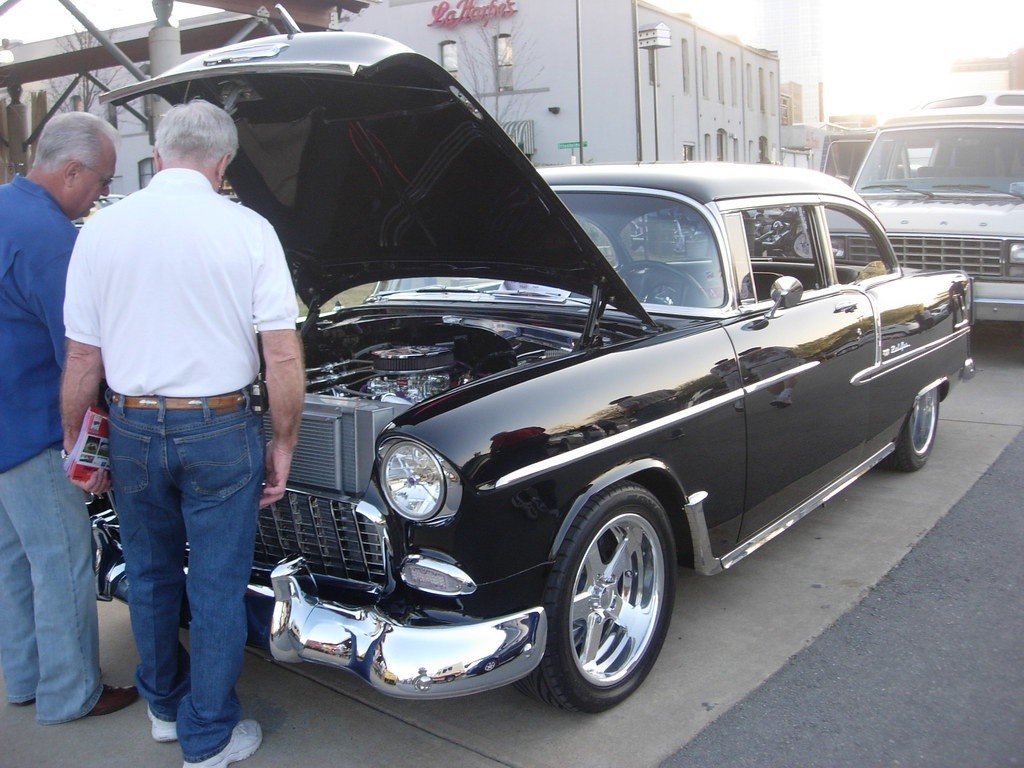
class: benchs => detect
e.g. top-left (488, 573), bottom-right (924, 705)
top-left (664, 261), bottom-right (861, 293)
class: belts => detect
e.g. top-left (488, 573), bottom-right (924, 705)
top-left (112, 392), bottom-right (250, 410)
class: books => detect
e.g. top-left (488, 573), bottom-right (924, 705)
top-left (64, 407), bottom-right (110, 483)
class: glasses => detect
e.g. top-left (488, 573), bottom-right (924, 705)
top-left (70, 160), bottom-right (113, 193)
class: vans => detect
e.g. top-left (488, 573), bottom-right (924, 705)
top-left (819, 109), bottom-right (1024, 323)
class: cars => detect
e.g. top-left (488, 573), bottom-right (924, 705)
top-left (84, 32), bottom-right (979, 713)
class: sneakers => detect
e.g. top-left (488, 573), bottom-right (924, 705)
top-left (183, 719), bottom-right (263, 768)
top-left (147, 702), bottom-right (179, 742)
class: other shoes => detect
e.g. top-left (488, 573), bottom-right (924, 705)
top-left (84, 684), bottom-right (138, 715)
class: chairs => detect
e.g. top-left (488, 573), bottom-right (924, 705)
top-left (740, 271), bottom-right (783, 306)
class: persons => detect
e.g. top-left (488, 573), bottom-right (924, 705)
top-left (1, 107), bottom-right (142, 724)
top-left (61, 99), bottom-right (304, 768)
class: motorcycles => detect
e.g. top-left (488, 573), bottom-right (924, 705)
top-left (629, 206), bottom-right (813, 259)
